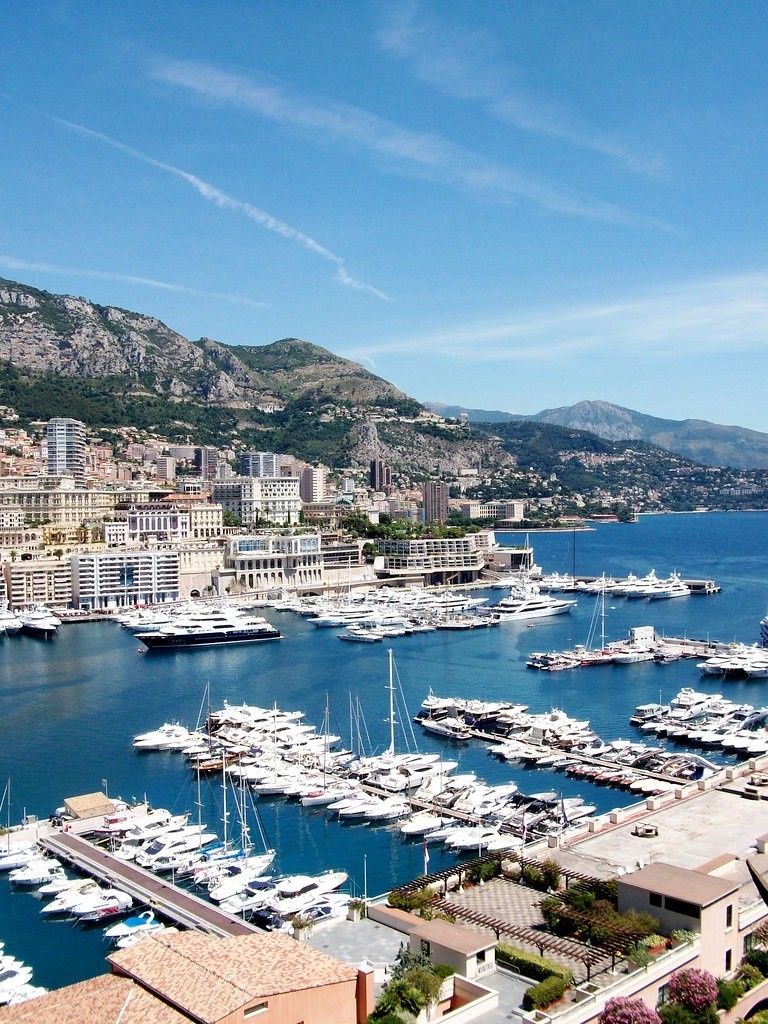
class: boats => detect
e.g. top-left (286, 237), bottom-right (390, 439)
top-left (0, 522), bottom-right (768, 1005)
